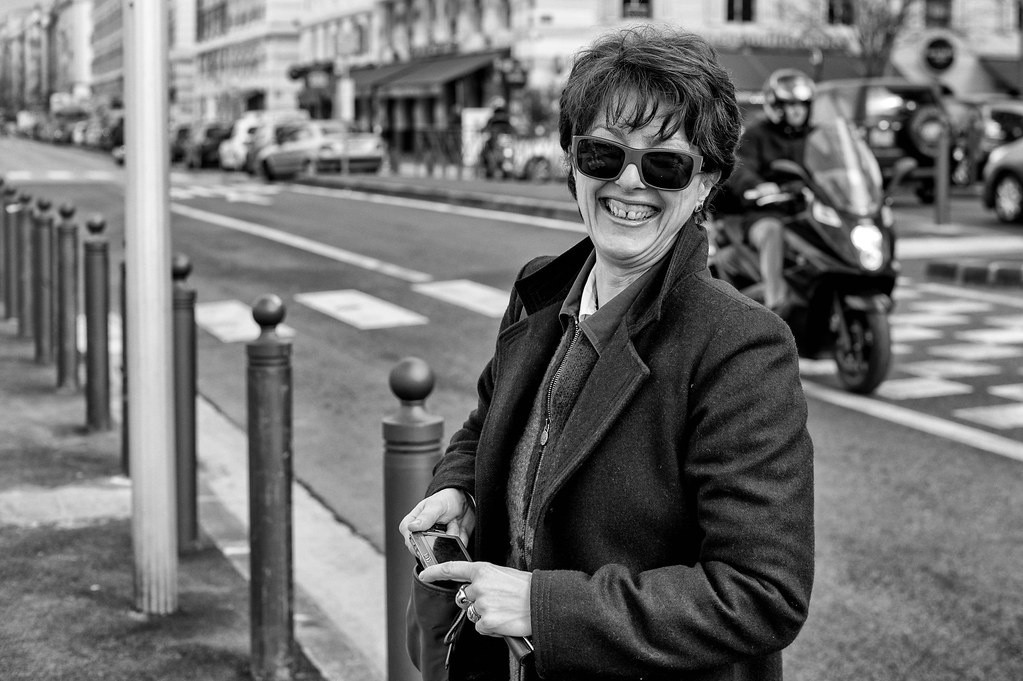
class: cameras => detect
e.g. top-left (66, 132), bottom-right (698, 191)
top-left (409, 530), bottom-right (473, 570)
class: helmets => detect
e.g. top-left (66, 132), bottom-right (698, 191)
top-left (763, 69), bottom-right (817, 125)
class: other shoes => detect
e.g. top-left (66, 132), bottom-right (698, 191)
top-left (766, 295), bottom-right (807, 320)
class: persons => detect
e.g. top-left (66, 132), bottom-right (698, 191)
top-left (399, 27), bottom-right (816, 681)
top-left (718, 68), bottom-right (820, 313)
top-left (480, 96), bottom-right (515, 167)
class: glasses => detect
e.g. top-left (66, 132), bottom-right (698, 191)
top-left (572, 120), bottom-right (716, 191)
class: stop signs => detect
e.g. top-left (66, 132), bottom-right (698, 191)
top-left (924, 36), bottom-right (954, 74)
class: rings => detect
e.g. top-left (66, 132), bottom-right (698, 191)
top-left (455, 584), bottom-right (470, 610)
top-left (467, 602), bottom-right (481, 622)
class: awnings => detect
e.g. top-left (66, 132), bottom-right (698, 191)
top-left (321, 56), bottom-right (443, 100)
top-left (298, 86), bottom-right (329, 107)
top-left (379, 52), bottom-right (510, 98)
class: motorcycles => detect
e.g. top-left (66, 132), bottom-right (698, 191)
top-left (477, 129), bottom-right (516, 184)
top-left (697, 112), bottom-right (916, 394)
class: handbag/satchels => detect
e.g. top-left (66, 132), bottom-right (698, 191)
top-left (406, 565), bottom-right (478, 681)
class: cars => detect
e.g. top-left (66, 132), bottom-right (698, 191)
top-left (500, 129), bottom-right (571, 185)
top-left (813, 75), bottom-right (1023, 228)
top-left (0, 83), bottom-right (313, 178)
top-left (249, 116), bottom-right (391, 188)
